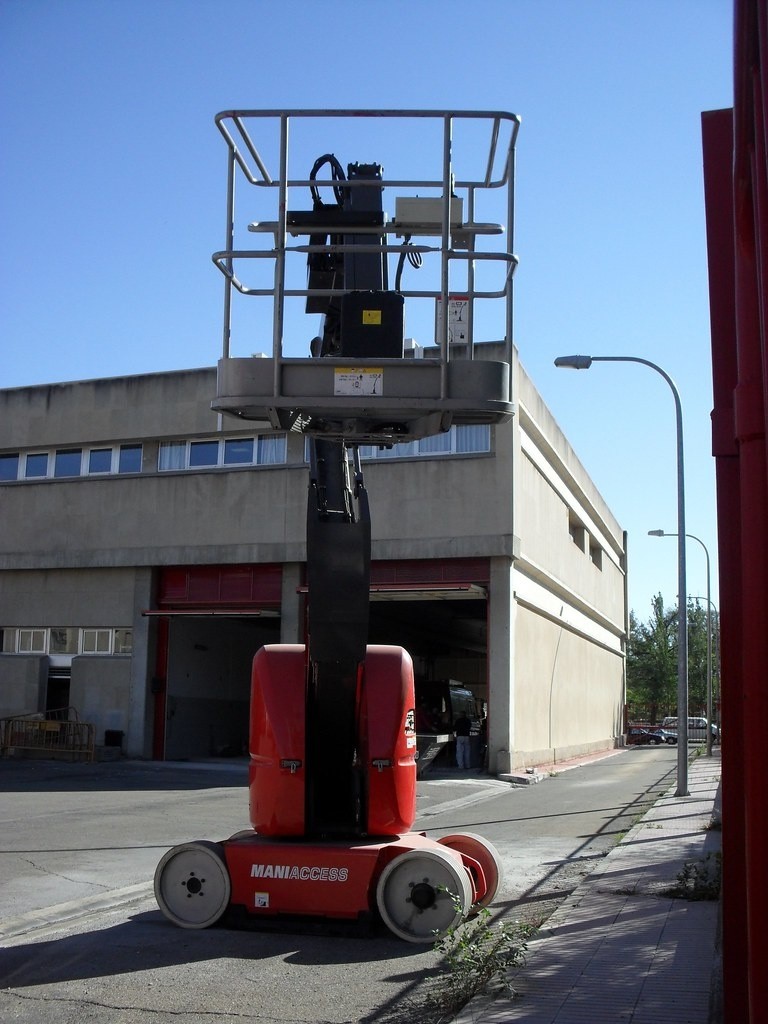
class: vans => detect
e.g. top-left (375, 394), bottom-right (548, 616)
top-left (662, 716), bottom-right (717, 743)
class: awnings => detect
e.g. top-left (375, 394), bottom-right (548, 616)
top-left (296, 582), bottom-right (488, 601)
top-left (141, 608), bottom-right (284, 618)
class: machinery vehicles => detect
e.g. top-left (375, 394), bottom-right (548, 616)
top-left (153, 153), bottom-right (504, 950)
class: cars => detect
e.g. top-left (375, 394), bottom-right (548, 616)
top-left (627, 724), bottom-right (678, 746)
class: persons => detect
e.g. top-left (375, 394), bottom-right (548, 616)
top-left (453, 710), bottom-right (472, 770)
top-left (414, 703), bottom-right (442, 734)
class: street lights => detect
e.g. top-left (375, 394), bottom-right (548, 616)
top-left (553, 354), bottom-right (692, 797)
top-left (675, 594), bottom-right (721, 746)
top-left (647, 529), bottom-right (712, 758)
